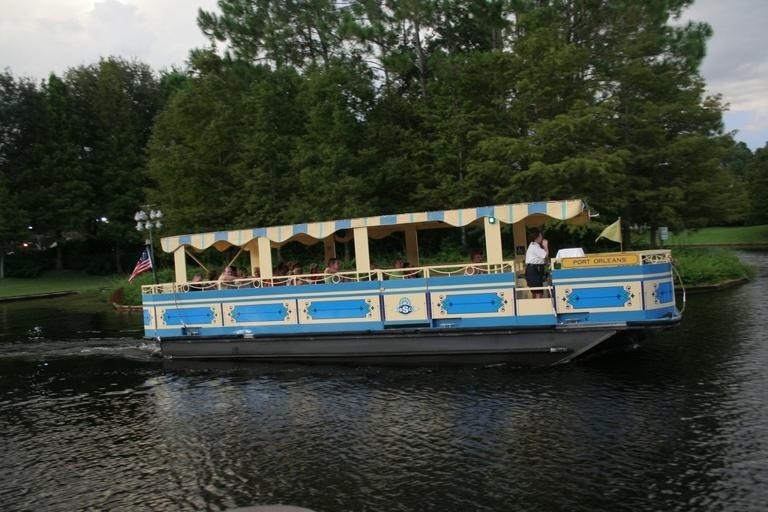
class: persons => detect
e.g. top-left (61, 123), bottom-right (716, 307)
top-left (462, 248), bottom-right (484, 275)
top-left (188, 257), bottom-right (419, 292)
top-left (525, 230), bottom-right (550, 299)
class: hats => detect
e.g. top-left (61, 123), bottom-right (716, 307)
top-left (287, 261), bottom-right (299, 271)
top-left (310, 263), bottom-right (318, 273)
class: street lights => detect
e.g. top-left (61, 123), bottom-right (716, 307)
top-left (133, 203), bottom-right (163, 281)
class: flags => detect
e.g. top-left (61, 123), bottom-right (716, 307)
top-left (127, 247), bottom-right (153, 286)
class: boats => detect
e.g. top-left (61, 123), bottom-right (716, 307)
top-left (140, 199), bottom-right (688, 365)
